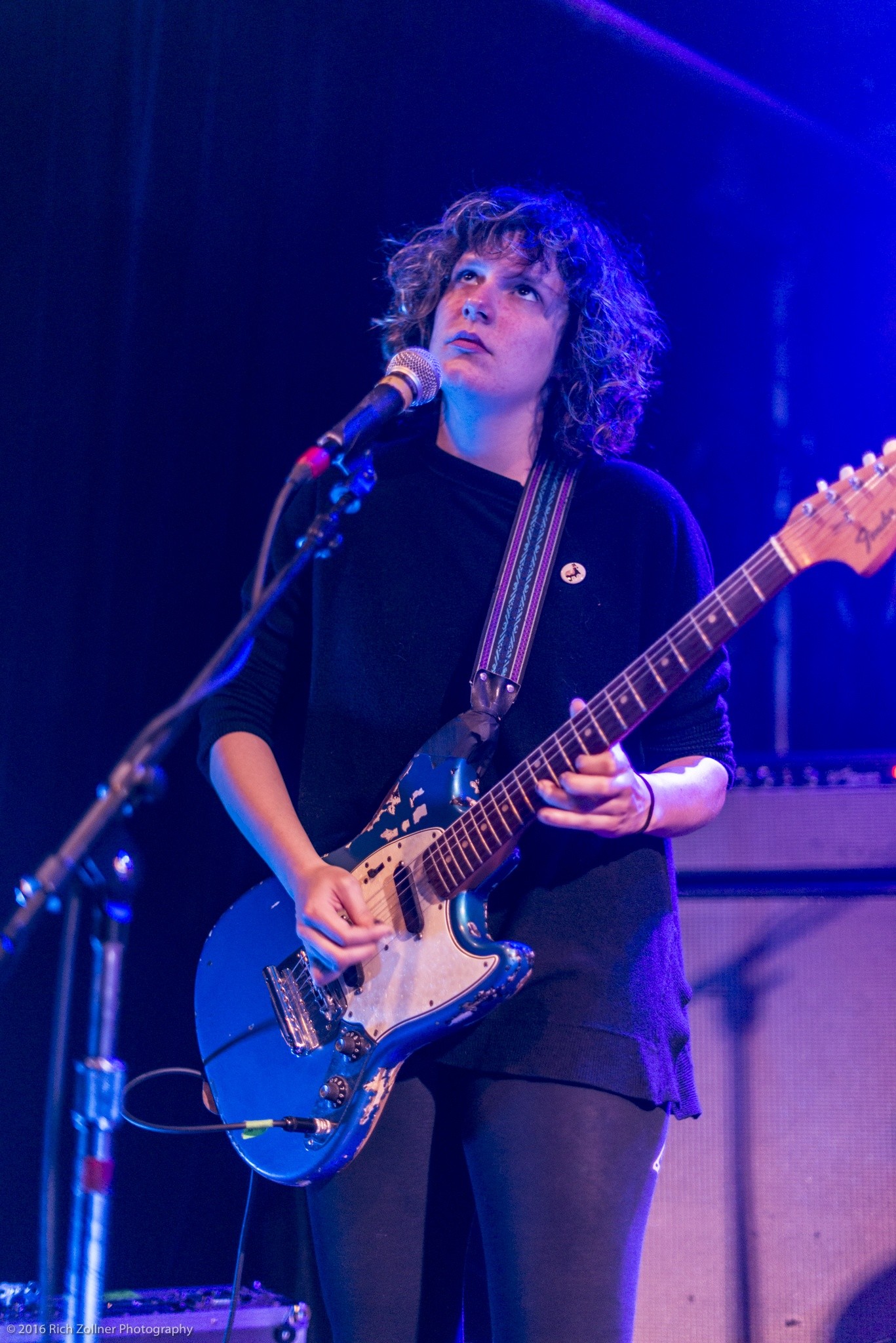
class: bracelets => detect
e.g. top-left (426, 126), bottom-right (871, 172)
top-left (634, 772), bottom-right (655, 833)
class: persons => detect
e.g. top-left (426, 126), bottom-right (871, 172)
top-left (196, 188), bottom-right (738, 1343)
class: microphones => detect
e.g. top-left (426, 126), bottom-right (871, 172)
top-left (288, 348), bottom-right (443, 487)
top-left (195, 1070), bottom-right (219, 1114)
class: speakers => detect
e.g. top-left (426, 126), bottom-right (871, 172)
top-left (633, 885), bottom-right (896, 1343)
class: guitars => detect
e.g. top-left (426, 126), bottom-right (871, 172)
top-left (193, 437), bottom-right (896, 1194)
